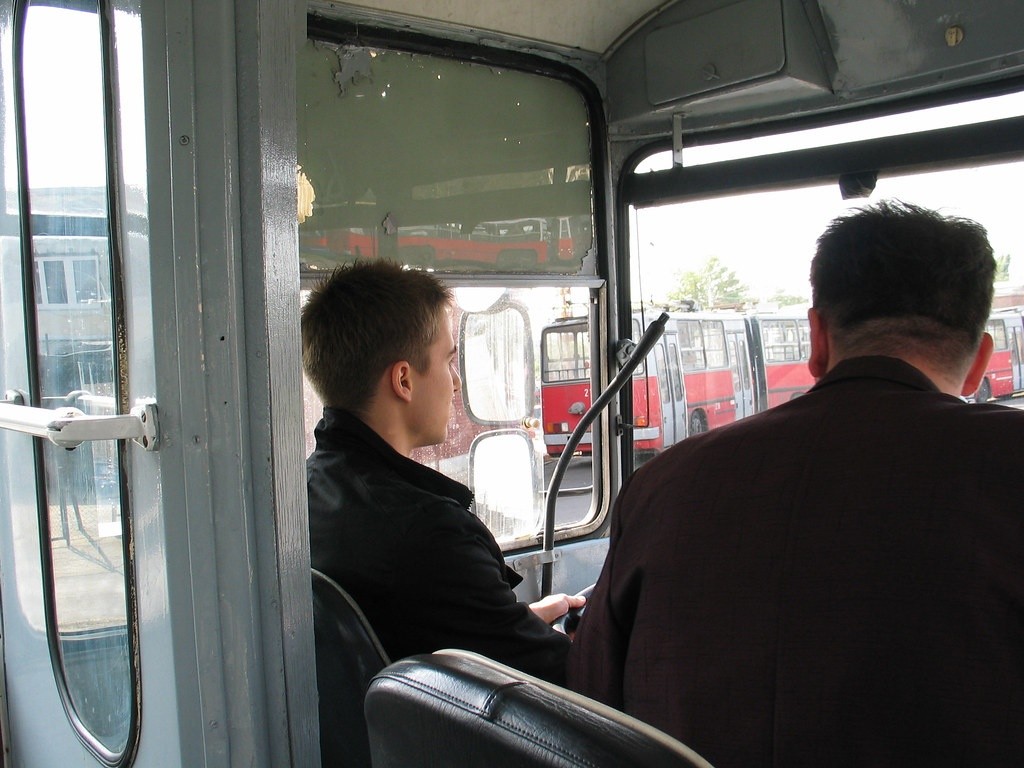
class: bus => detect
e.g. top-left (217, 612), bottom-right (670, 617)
top-left (301, 218), bottom-right (585, 269)
top-left (537, 299), bottom-right (1024, 464)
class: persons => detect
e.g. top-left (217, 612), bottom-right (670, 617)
top-left (301, 257), bottom-right (588, 689)
top-left (565, 196), bottom-right (1023, 768)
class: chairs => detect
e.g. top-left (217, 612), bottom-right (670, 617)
top-left (303, 563), bottom-right (409, 768)
top-left (361, 644), bottom-right (712, 768)
